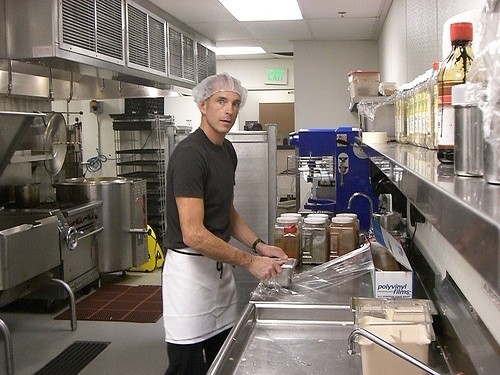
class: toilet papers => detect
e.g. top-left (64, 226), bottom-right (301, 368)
top-left (362, 131), bottom-right (387, 144)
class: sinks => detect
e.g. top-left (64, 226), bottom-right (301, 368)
top-left (205, 304), bottom-right (453, 375)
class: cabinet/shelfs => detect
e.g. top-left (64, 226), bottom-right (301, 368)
top-left (112, 116), bottom-right (175, 250)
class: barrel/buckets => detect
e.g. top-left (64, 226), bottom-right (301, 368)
top-left (52, 178), bottom-right (134, 276)
top-left (14, 183), bottom-right (41, 208)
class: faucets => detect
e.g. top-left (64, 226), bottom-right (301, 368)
top-left (347, 192), bottom-right (374, 235)
top-left (347, 327), bottom-right (445, 375)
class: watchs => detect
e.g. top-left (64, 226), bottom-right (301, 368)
top-left (252, 238), bottom-right (266, 253)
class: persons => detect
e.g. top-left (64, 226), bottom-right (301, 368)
top-left (161, 72), bottom-right (289, 375)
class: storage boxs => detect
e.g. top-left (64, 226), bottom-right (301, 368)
top-left (348, 70), bottom-right (380, 101)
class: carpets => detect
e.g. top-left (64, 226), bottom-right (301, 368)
top-left (53, 285), bottom-right (163, 323)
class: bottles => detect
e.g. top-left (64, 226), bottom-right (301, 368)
top-left (393, 20), bottom-right (477, 165)
top-left (273, 212), bottom-right (361, 267)
